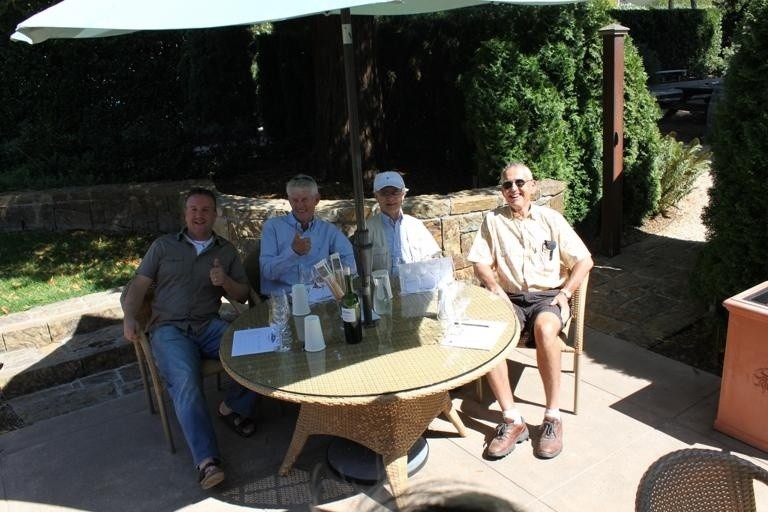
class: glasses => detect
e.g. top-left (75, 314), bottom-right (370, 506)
top-left (502, 179), bottom-right (532, 189)
top-left (380, 190), bottom-right (401, 196)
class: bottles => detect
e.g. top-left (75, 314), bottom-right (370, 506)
top-left (373, 276), bottom-right (394, 314)
top-left (374, 317), bottom-right (394, 354)
top-left (340, 267), bottom-right (363, 344)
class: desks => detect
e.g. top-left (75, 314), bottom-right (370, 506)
top-left (219, 276), bottom-right (521, 509)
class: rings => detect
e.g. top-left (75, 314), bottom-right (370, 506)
top-left (214, 276), bottom-right (217, 283)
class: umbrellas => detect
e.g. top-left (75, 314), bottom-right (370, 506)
top-left (8, 0), bottom-right (586, 329)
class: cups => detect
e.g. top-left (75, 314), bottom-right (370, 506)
top-left (305, 315), bottom-right (326, 354)
top-left (373, 269), bottom-right (394, 300)
top-left (303, 351), bottom-right (326, 377)
top-left (291, 284), bottom-right (311, 316)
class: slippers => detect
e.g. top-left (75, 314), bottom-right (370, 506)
top-left (199, 462), bottom-right (224, 490)
top-left (217, 400), bottom-right (256, 436)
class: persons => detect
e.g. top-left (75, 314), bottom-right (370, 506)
top-left (466, 161), bottom-right (595, 460)
top-left (353, 169), bottom-right (443, 279)
top-left (259, 173), bottom-right (358, 298)
top-left (120, 187), bottom-right (258, 491)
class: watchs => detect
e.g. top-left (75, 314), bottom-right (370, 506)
top-left (559, 287), bottom-right (572, 299)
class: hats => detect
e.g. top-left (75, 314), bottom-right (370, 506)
top-left (373, 171), bottom-right (405, 192)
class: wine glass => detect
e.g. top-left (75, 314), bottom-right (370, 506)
top-left (268, 288), bottom-right (292, 353)
top-left (298, 263), bottom-right (329, 301)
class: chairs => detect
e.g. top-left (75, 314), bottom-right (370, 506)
top-left (475, 272), bottom-right (590, 415)
top-left (635, 448), bottom-right (768, 512)
top-left (242, 236), bottom-right (262, 308)
top-left (120, 279), bottom-right (245, 455)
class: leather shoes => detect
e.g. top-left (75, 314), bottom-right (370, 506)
top-left (536, 416), bottom-right (562, 458)
top-left (488, 416), bottom-right (529, 458)
